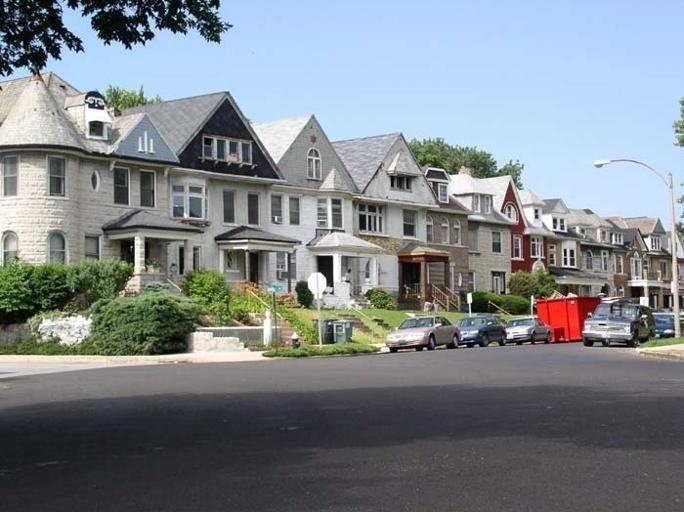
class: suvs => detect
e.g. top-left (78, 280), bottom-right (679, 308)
top-left (580, 300), bottom-right (684, 350)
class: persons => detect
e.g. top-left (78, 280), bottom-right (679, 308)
top-left (344, 268), bottom-right (353, 296)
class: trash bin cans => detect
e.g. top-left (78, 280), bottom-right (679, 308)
top-left (312, 318), bottom-right (354, 344)
top-left (274, 283), bottom-right (283, 291)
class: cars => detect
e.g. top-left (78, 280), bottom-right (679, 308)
top-left (385, 315), bottom-right (461, 352)
top-left (454, 316), bottom-right (507, 348)
top-left (502, 317), bottom-right (552, 346)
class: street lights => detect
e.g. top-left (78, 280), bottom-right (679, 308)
top-left (593, 158), bottom-right (681, 340)
